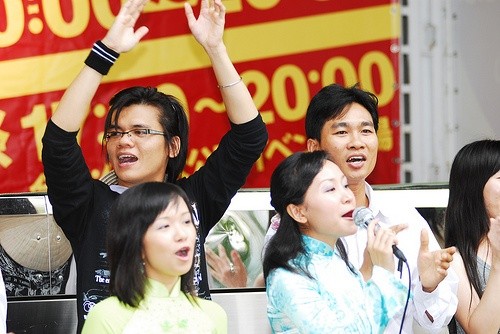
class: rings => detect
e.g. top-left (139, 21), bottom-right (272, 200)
top-left (227, 263), bottom-right (236, 272)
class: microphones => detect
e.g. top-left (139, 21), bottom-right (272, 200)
top-left (353, 206), bottom-right (406, 261)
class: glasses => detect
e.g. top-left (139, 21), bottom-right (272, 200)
top-left (103, 128), bottom-right (164, 142)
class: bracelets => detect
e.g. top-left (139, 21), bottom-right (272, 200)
top-left (214, 76), bottom-right (243, 89)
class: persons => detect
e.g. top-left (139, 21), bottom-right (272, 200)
top-left (0, 198), bottom-right (73, 296)
top-left (263, 148), bottom-right (413, 334)
top-left (204, 213), bottom-right (281, 288)
top-left (443, 138), bottom-right (500, 334)
top-left (40, 0), bottom-right (269, 334)
top-left (0, 268), bottom-right (16, 334)
top-left (79, 180), bottom-right (230, 334)
top-left (306, 81), bottom-right (459, 334)
